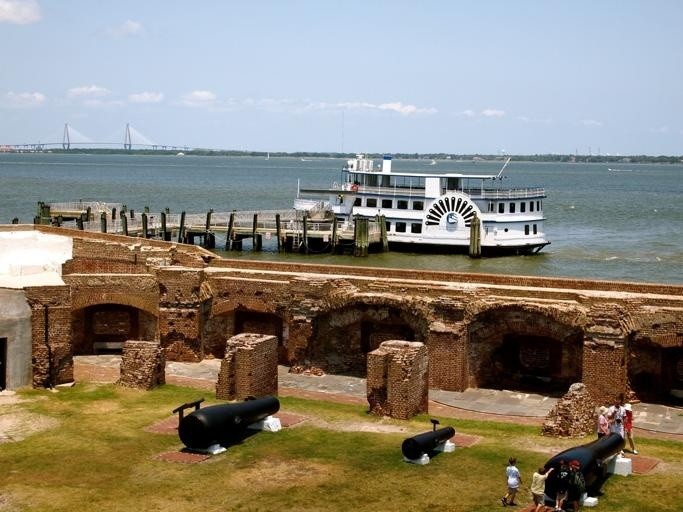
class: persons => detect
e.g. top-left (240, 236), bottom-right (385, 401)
top-left (554, 460), bottom-right (570, 512)
top-left (568, 460), bottom-right (581, 512)
top-left (501, 457), bottom-right (523, 507)
top-left (618, 393), bottom-right (640, 454)
top-left (351, 181), bottom-right (358, 193)
top-left (530, 468), bottom-right (554, 512)
top-left (597, 406), bottom-right (612, 439)
top-left (606, 397), bottom-right (627, 457)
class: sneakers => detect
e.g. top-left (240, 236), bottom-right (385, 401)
top-left (501, 497), bottom-right (517, 507)
top-left (632, 450), bottom-right (638, 454)
top-left (554, 507), bottom-right (562, 512)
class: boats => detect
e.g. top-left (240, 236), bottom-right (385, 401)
top-left (291, 152), bottom-right (551, 253)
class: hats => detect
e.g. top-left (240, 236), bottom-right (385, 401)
top-left (600, 406), bottom-right (609, 415)
